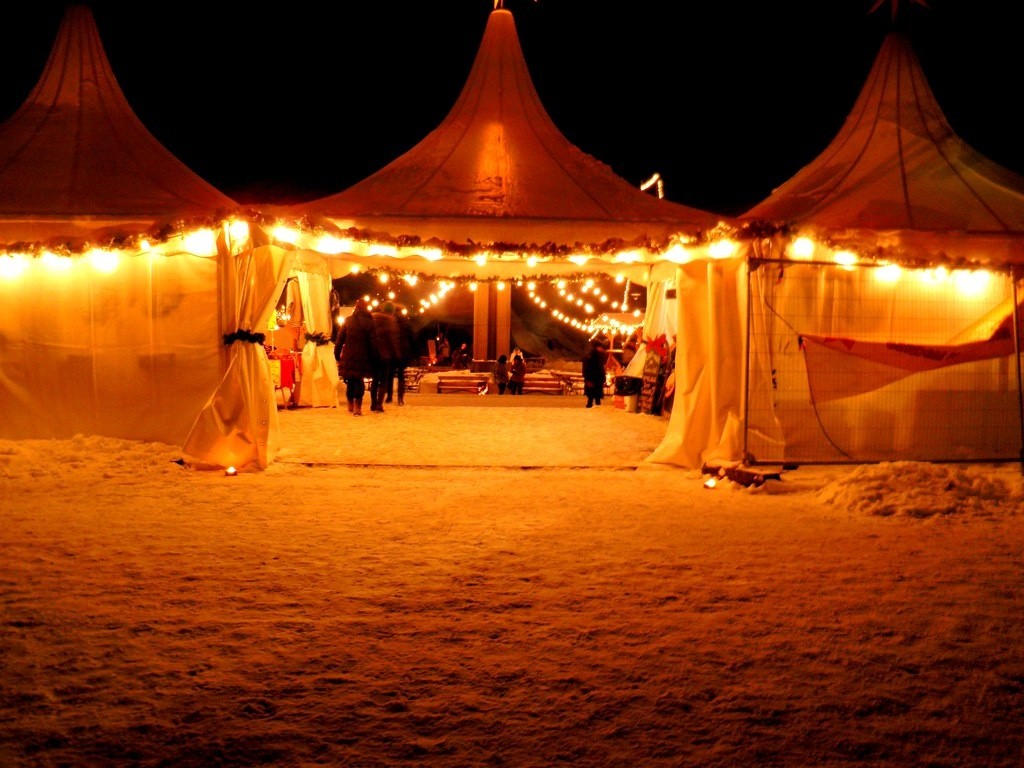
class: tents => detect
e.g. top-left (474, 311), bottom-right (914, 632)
top-left (244, 8), bottom-right (744, 408)
top-left (0, 0), bottom-right (277, 467)
top-left (636, 25), bottom-right (1023, 469)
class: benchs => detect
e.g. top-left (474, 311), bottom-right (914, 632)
top-left (516, 377), bottom-right (563, 395)
top-left (436, 375), bottom-right (491, 395)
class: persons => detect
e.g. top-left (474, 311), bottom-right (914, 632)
top-left (493, 354), bottom-right (510, 395)
top-left (385, 305), bottom-right (418, 406)
top-left (333, 298), bottom-right (380, 417)
top-left (434, 335), bottom-right (469, 370)
top-left (507, 354), bottom-right (527, 396)
top-left (582, 324), bottom-right (641, 409)
top-left (369, 301), bottom-right (400, 412)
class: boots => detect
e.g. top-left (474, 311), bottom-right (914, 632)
top-left (354, 398), bottom-right (362, 416)
top-left (396, 381), bottom-right (405, 406)
top-left (348, 397), bottom-right (354, 412)
top-left (385, 384), bottom-right (392, 403)
top-left (371, 386), bottom-right (386, 411)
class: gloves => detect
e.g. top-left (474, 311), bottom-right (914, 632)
top-left (335, 353), bottom-right (340, 361)
